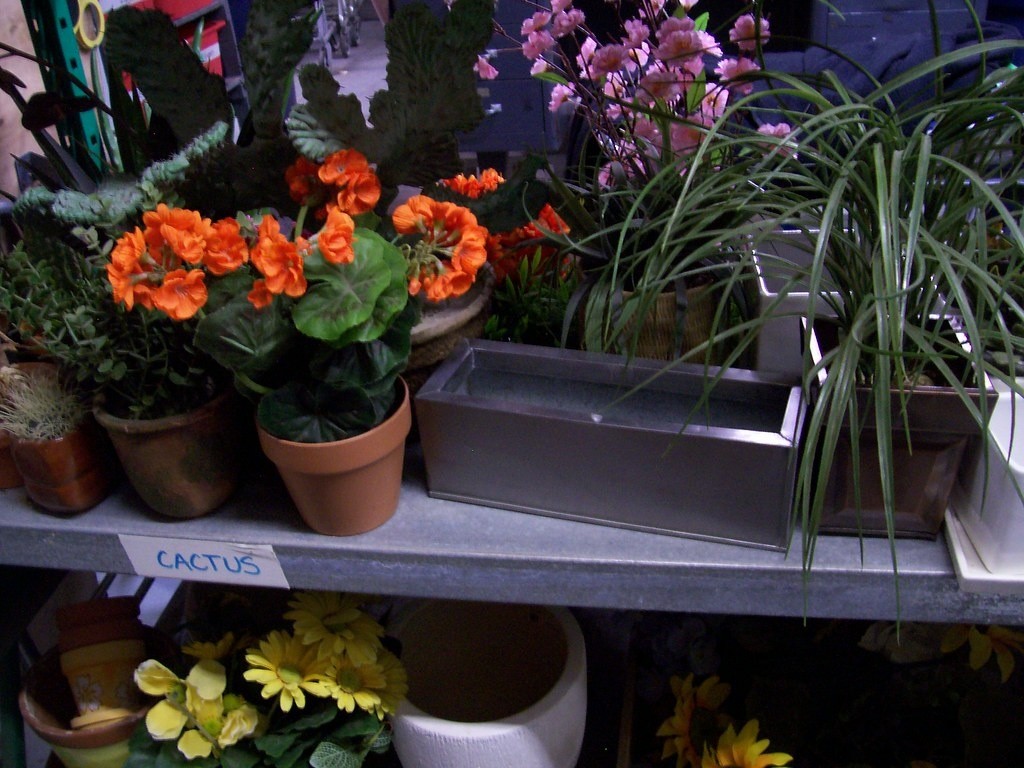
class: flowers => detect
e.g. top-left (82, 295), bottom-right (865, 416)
top-left (104, 147), bottom-right (490, 443)
top-left (122, 584), bottom-right (408, 768)
top-left (857, 620), bottom-right (1024, 684)
top-left (471, 0), bottom-right (800, 292)
top-left (654, 671), bottom-right (795, 768)
top-left (435, 166), bottom-right (571, 286)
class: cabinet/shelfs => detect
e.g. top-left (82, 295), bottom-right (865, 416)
top-left (90, 0), bottom-right (250, 140)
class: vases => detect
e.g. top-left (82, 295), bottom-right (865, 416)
top-left (580, 272), bottom-right (717, 365)
top-left (385, 600), bottom-right (587, 768)
top-left (18, 624), bottom-right (183, 768)
top-left (255, 374), bottom-right (412, 537)
top-left (745, 228), bottom-right (854, 377)
top-left (492, 263), bottom-right (572, 343)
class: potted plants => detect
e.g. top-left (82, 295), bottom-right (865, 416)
top-left (0, 368), bottom-right (118, 514)
top-left (0, 226), bottom-right (247, 519)
top-left (298, 0), bottom-right (510, 447)
top-left (520, 0), bottom-right (1024, 647)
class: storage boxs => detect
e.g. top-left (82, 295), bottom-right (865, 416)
top-left (180, 18), bottom-right (226, 76)
top-left (153, 0), bottom-right (213, 22)
